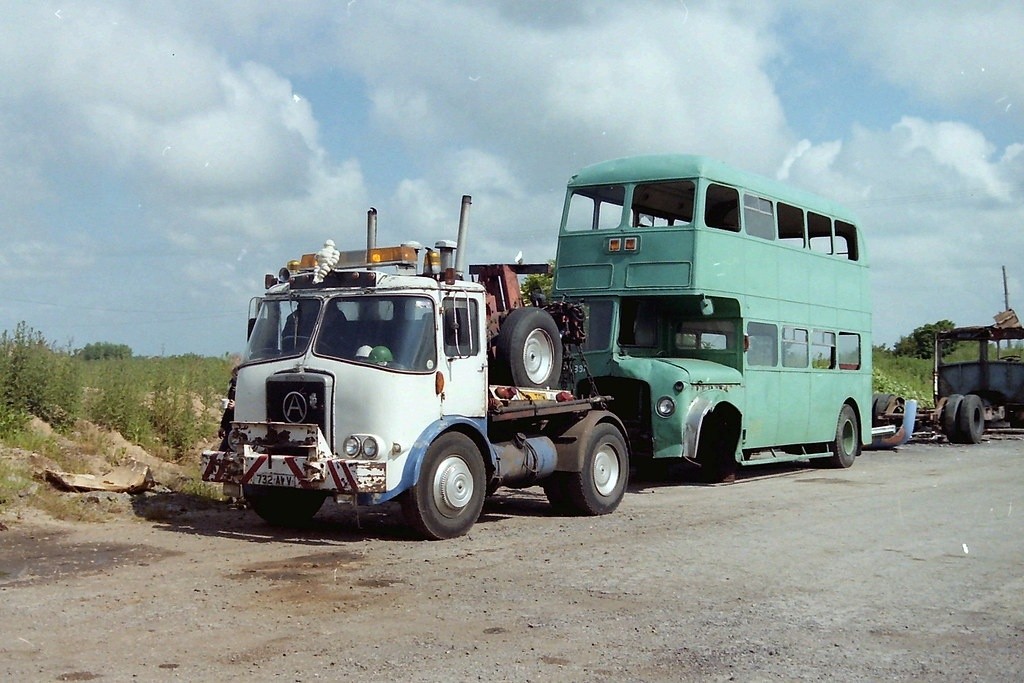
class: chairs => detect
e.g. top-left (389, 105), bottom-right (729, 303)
top-left (280, 298), bottom-right (347, 357)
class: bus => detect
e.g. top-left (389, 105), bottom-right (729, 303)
top-left (554, 151), bottom-right (873, 482)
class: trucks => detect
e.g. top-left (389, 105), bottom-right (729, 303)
top-left (201, 196), bottom-right (631, 540)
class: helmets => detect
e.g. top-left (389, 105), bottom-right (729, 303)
top-left (365, 345), bottom-right (393, 363)
top-left (355, 344), bottom-right (372, 358)
